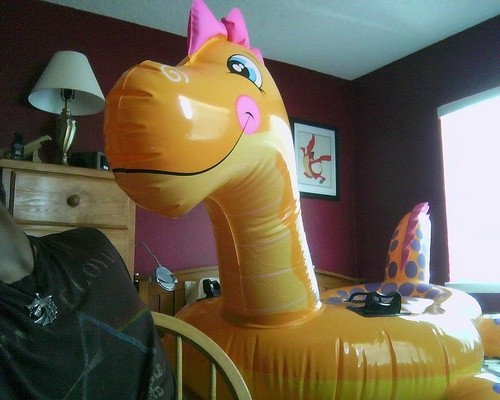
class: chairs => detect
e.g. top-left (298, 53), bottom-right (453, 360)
top-left (151, 311), bottom-right (253, 400)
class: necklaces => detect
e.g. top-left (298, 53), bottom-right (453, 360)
top-left (5, 232), bottom-right (57, 326)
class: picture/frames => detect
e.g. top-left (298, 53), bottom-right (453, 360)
top-left (289, 117), bottom-right (339, 201)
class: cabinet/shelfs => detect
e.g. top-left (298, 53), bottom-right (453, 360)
top-left (0, 158), bottom-right (137, 287)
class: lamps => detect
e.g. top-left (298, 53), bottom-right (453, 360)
top-left (27, 50), bottom-right (106, 164)
top-left (135, 241), bottom-right (178, 292)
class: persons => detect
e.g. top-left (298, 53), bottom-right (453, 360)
top-left (0, 204), bottom-right (176, 400)
